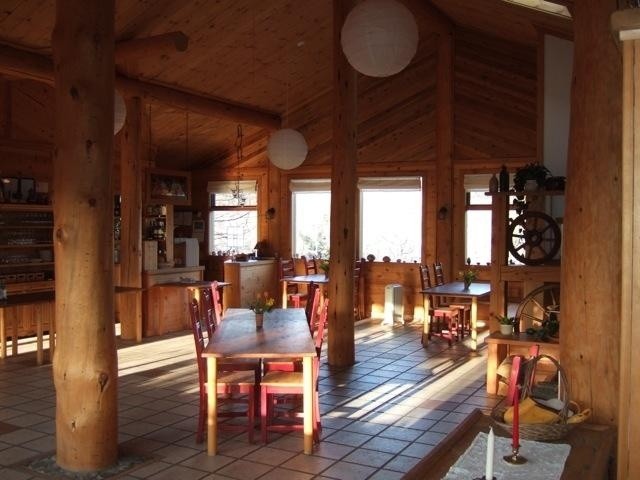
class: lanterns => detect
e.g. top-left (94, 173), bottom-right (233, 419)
top-left (341, 0), bottom-right (419, 78)
top-left (114, 89), bottom-right (126, 136)
top-left (266, 128), bottom-right (308, 170)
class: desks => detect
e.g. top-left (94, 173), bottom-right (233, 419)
top-left (399, 408), bottom-right (617, 480)
top-left (484, 330), bottom-right (560, 399)
top-left (419, 281), bottom-right (491, 350)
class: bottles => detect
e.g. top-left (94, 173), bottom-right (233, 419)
top-left (489, 166), bottom-right (509, 193)
top-left (147, 220), bottom-right (164, 239)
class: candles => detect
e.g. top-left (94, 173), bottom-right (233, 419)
top-left (513, 384), bottom-right (518, 446)
top-left (485, 426), bottom-right (494, 480)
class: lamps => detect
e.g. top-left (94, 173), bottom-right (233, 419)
top-left (341, 1), bottom-right (419, 78)
top-left (267, 57), bottom-right (308, 170)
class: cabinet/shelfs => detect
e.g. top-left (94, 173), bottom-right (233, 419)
top-left (485, 188), bottom-right (563, 330)
top-left (0, 174), bottom-right (55, 340)
top-left (111, 193), bottom-right (174, 323)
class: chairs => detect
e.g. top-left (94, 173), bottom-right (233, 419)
top-left (419, 264), bottom-right (460, 347)
top-left (184, 280), bottom-right (330, 448)
top-left (433, 263), bottom-right (471, 340)
top-left (277, 254), bottom-right (366, 321)
top-left (507, 344), bottom-right (541, 408)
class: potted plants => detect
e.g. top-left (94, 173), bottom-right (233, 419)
top-left (458, 269), bottom-right (476, 290)
top-left (490, 311), bottom-right (518, 335)
